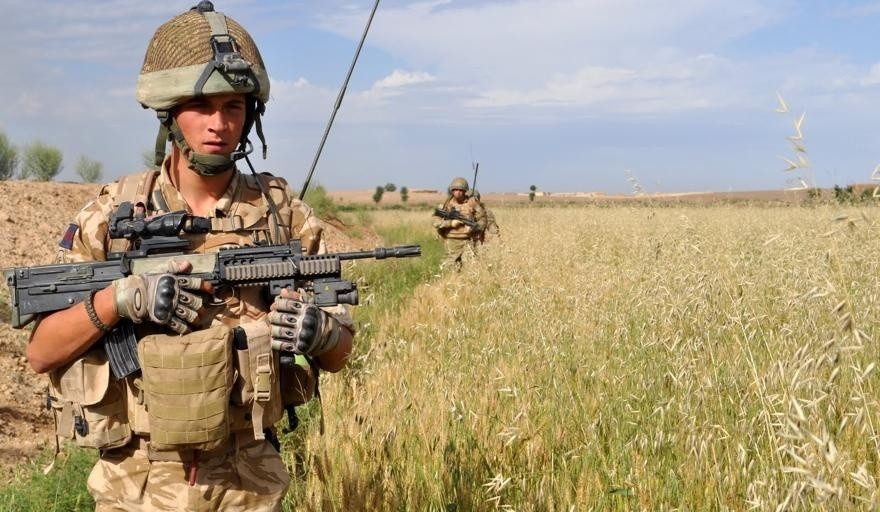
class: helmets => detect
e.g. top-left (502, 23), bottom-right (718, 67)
top-left (132, 4), bottom-right (272, 109)
top-left (465, 189), bottom-right (481, 203)
top-left (449, 177), bottom-right (470, 192)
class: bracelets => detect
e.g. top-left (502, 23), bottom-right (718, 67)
top-left (84, 288), bottom-right (118, 333)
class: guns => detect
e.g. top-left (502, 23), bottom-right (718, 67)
top-left (434, 206), bottom-right (480, 229)
top-left (2, 202), bottom-right (422, 381)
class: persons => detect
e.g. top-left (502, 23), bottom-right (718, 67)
top-left (467, 190), bottom-right (500, 251)
top-left (434, 178), bottom-right (487, 270)
top-left (25, 1), bottom-right (353, 511)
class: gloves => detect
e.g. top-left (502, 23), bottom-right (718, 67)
top-left (464, 225), bottom-right (472, 234)
top-left (451, 219), bottom-right (464, 228)
top-left (268, 286), bottom-right (342, 360)
top-left (110, 258), bottom-right (206, 336)
